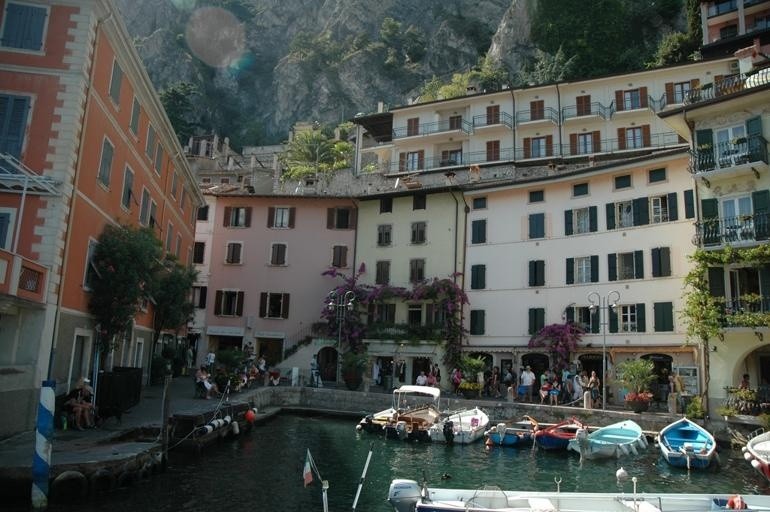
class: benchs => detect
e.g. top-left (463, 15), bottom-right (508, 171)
top-left (536, 385), bottom-right (563, 404)
top-left (54, 392), bottom-right (73, 430)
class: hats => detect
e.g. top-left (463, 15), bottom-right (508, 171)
top-left (525, 366), bottom-right (532, 370)
top-left (84, 385), bottom-right (94, 396)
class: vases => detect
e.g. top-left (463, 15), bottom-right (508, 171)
top-left (463, 390), bottom-right (477, 398)
top-left (627, 401), bottom-right (650, 413)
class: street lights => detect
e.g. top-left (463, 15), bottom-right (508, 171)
top-left (327, 289), bottom-right (356, 388)
top-left (586, 290), bottom-right (621, 408)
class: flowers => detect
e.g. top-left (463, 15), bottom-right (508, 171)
top-left (624, 392), bottom-right (654, 401)
top-left (458, 383), bottom-right (483, 392)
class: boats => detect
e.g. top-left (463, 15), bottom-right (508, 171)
top-left (740, 426), bottom-right (770, 482)
top-left (529, 416), bottom-right (586, 450)
top-left (484, 412), bottom-right (538, 446)
top-left (569, 417), bottom-right (645, 456)
top-left (429, 405), bottom-right (490, 445)
top-left (385, 404), bottom-right (441, 442)
top-left (654, 457), bottom-right (711, 493)
top-left (168, 378), bottom-right (256, 453)
top-left (387, 469), bottom-right (770, 512)
top-left (656, 415), bottom-right (717, 467)
top-left (354, 404), bottom-right (401, 434)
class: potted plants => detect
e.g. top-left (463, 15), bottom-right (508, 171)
top-left (151, 353), bottom-right (172, 385)
top-left (339, 352), bottom-right (369, 391)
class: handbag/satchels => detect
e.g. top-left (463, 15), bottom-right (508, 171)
top-left (493, 388), bottom-right (502, 398)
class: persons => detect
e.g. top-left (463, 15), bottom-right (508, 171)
top-left (668, 370), bottom-right (685, 410)
top-left (416, 363), bottom-right (461, 395)
top-left (739, 373), bottom-right (751, 411)
top-left (476, 365), bottom-right (602, 407)
top-left (187, 346), bottom-right (266, 400)
top-left (62, 378), bottom-right (97, 432)
